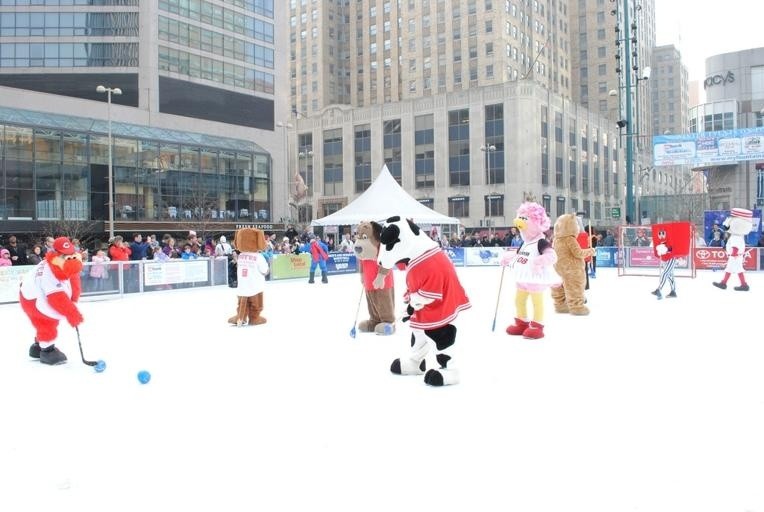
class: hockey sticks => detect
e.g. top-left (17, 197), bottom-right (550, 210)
top-left (76, 325), bottom-right (97, 366)
top-left (656, 252), bottom-right (663, 300)
top-left (491, 265), bottom-right (505, 332)
top-left (587, 220), bottom-right (596, 279)
top-left (350, 282), bottom-right (365, 339)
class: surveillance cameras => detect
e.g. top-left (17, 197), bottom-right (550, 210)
top-left (643, 66), bottom-right (652, 80)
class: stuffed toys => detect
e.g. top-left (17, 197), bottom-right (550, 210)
top-left (576, 216), bottom-right (591, 291)
top-left (375, 214), bottom-right (473, 387)
top-left (352, 219), bottom-right (398, 335)
top-left (500, 201), bottom-right (563, 340)
top-left (17, 235), bottom-right (84, 365)
top-left (650, 221), bottom-right (695, 299)
top-left (712, 207), bottom-right (754, 292)
top-left (227, 226), bottom-right (270, 325)
top-left (552, 212), bottom-right (597, 315)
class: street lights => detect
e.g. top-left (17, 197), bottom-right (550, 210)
top-left (608, 0), bottom-right (650, 229)
top-left (480, 142), bottom-right (497, 245)
top-left (96, 84), bottom-right (124, 243)
top-left (276, 121), bottom-right (292, 232)
top-left (298, 150), bottom-right (312, 228)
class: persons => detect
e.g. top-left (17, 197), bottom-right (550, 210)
top-left (1, 233), bottom-right (56, 265)
top-left (72, 231), bottom-right (232, 292)
top-left (300, 234), bottom-right (329, 283)
top-left (438, 224), bottom-right (726, 246)
top-left (264, 231), bottom-right (353, 254)
